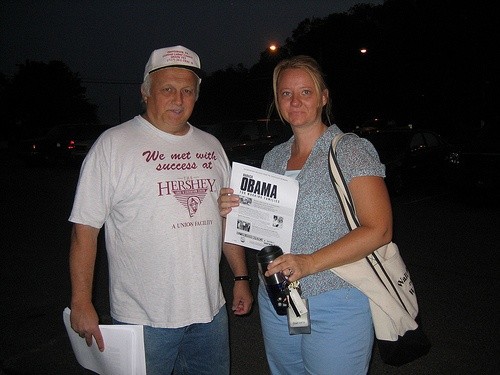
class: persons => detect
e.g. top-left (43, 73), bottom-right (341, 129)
top-left (66, 44), bottom-right (254, 375)
top-left (217, 57), bottom-right (394, 374)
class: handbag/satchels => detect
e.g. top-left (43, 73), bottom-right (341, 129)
top-left (328, 242), bottom-right (419, 342)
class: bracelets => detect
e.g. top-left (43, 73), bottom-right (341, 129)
top-left (233, 275), bottom-right (250, 282)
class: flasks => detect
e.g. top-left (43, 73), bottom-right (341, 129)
top-left (256, 245), bottom-right (289, 316)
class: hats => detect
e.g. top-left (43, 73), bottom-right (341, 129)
top-left (144, 45), bottom-right (207, 82)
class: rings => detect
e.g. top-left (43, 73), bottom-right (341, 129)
top-left (287, 268), bottom-right (293, 275)
